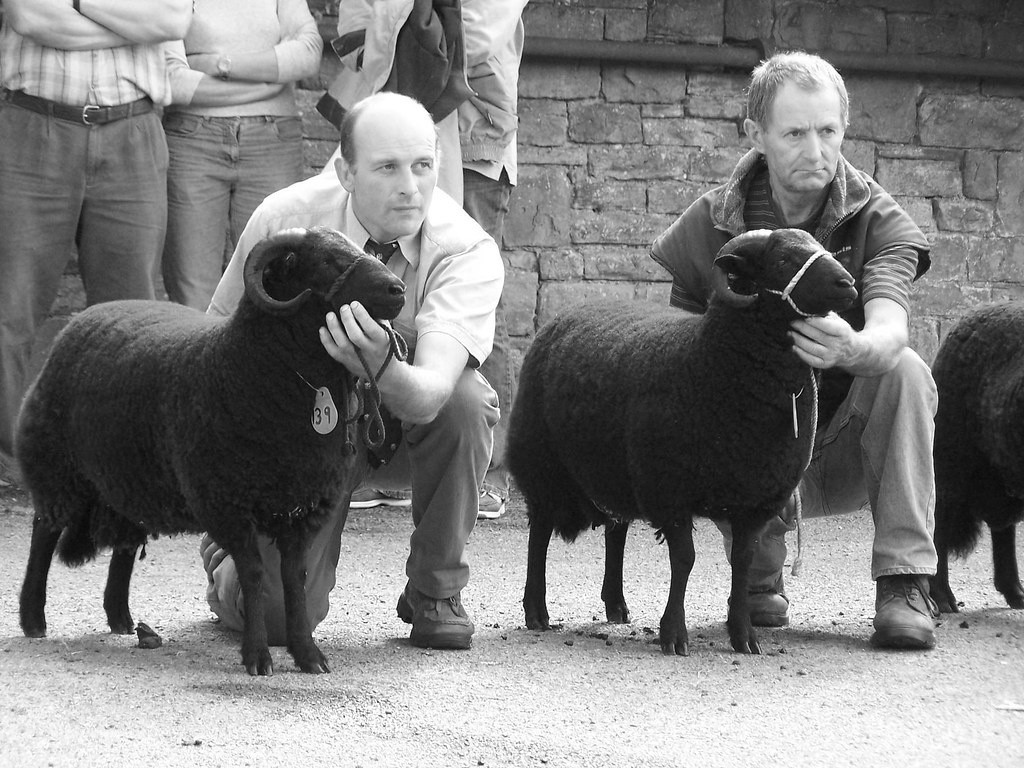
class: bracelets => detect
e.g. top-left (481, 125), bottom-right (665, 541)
top-left (73, 0), bottom-right (79, 12)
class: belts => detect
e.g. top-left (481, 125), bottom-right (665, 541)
top-left (3, 89), bottom-right (153, 126)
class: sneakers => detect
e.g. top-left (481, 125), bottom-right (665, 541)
top-left (727, 568), bottom-right (790, 626)
top-left (478, 489), bottom-right (506, 518)
top-left (396, 577), bottom-right (475, 649)
top-left (349, 485), bottom-right (412, 508)
top-left (872, 574), bottom-right (940, 649)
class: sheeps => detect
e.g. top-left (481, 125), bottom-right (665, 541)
top-left (12, 224), bottom-right (407, 677)
top-left (504, 228), bottom-right (859, 656)
top-left (928, 301), bottom-right (1024, 614)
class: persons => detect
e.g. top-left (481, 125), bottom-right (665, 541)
top-left (199, 92), bottom-right (504, 649)
top-left (319, 0), bottom-right (530, 518)
top-left (0, 0), bottom-right (193, 487)
top-left (650, 52), bottom-right (939, 646)
top-left (163, 0), bottom-right (324, 313)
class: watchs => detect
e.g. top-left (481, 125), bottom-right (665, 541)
top-left (216, 55), bottom-right (233, 79)
